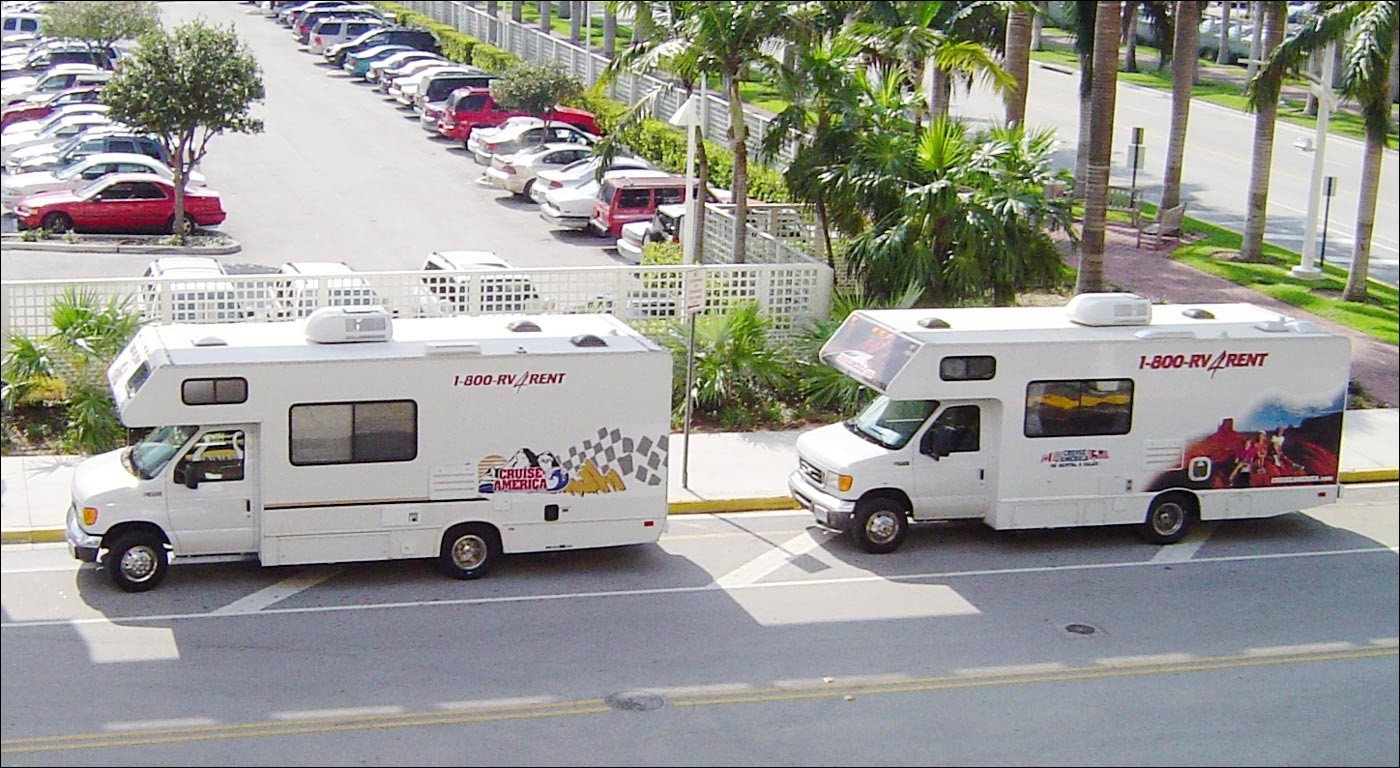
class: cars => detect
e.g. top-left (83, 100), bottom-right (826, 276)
top-left (256, 0), bottom-right (734, 232)
top-left (616, 220), bottom-right (653, 260)
top-left (13, 173), bottom-right (227, 238)
top-left (1, 1), bottom-right (209, 210)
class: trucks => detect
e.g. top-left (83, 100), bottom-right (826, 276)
top-left (61, 305), bottom-right (671, 592)
top-left (788, 292), bottom-right (1353, 553)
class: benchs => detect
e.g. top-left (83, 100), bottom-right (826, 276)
top-left (1136, 202), bottom-right (1187, 251)
top-left (1070, 180), bottom-right (1143, 227)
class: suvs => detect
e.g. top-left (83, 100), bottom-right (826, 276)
top-left (642, 204), bottom-right (806, 251)
top-left (136, 259), bottom-right (254, 322)
top-left (590, 176), bottom-right (767, 237)
top-left (267, 261), bottom-right (400, 319)
top-left (417, 252), bottom-right (540, 321)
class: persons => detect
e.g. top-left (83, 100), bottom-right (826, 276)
top-left (1228, 425), bottom-right (1305, 487)
